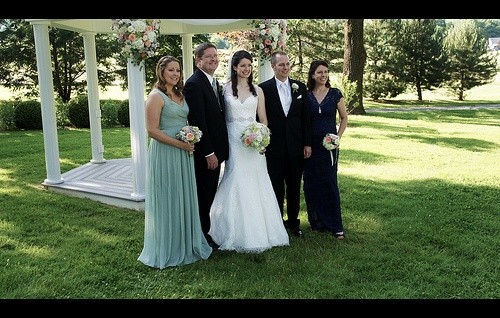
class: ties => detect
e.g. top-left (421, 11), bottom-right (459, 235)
top-left (212, 78), bottom-right (219, 98)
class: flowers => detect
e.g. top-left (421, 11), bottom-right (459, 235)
top-left (292, 83), bottom-right (299, 92)
top-left (244, 19), bottom-right (288, 66)
top-left (241, 122), bottom-right (271, 156)
top-left (176, 126), bottom-right (203, 157)
top-left (323, 133), bottom-right (340, 151)
top-left (111, 19), bottom-right (161, 71)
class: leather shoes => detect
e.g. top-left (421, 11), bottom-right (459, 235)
top-left (291, 227), bottom-right (302, 235)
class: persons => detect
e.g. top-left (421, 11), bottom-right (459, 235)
top-left (207, 50), bottom-right (290, 254)
top-left (183, 44), bottom-right (228, 245)
top-left (136, 56), bottom-right (213, 270)
top-left (301, 60), bottom-right (348, 239)
top-left (256, 51), bottom-right (312, 235)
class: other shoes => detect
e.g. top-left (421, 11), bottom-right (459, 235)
top-left (335, 231), bottom-right (345, 240)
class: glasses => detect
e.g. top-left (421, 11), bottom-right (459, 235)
top-left (205, 54), bottom-right (218, 58)
